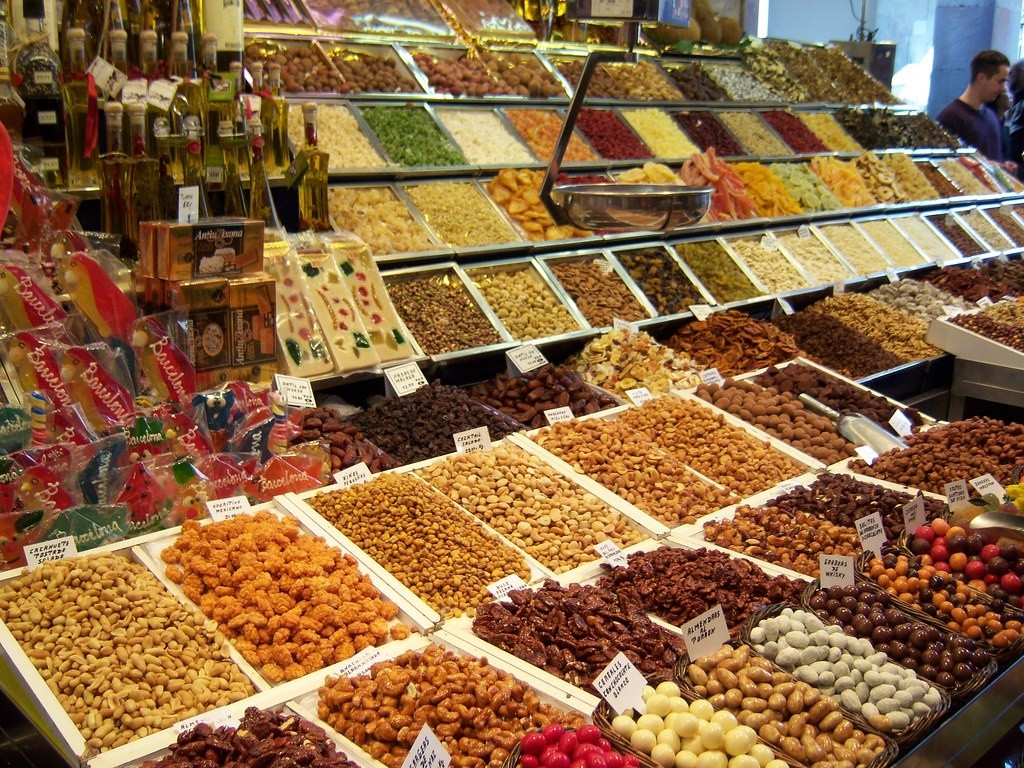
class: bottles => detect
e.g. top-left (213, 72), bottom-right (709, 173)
top-left (182, 115), bottom-right (214, 219)
top-left (171, 32), bottom-right (211, 178)
top-left (224, 61), bottom-right (250, 177)
top-left (219, 121), bottom-right (248, 217)
top-left (128, 103), bottom-right (164, 267)
top-left (268, 64), bottom-right (290, 175)
top-left (251, 63), bottom-right (274, 176)
top-left (248, 120), bottom-right (282, 242)
top-left (202, 33), bottom-right (233, 183)
top-left (63, 28), bottom-right (97, 186)
top-left (96, 102), bottom-right (127, 267)
top-left (15, 0), bottom-right (67, 189)
top-left (141, 32), bottom-right (170, 180)
top-left (108, 30), bottom-right (130, 101)
top-left (0, 12), bottom-right (22, 156)
top-left (154, 117), bottom-right (179, 221)
top-left (298, 102), bottom-right (329, 233)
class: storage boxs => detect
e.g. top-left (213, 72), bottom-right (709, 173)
top-left (0, 0), bottom-right (1024, 768)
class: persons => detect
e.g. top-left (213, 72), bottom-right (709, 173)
top-left (936, 50), bottom-right (1024, 185)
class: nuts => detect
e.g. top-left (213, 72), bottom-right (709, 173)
top-left (2, 34), bottom-right (1023, 768)
